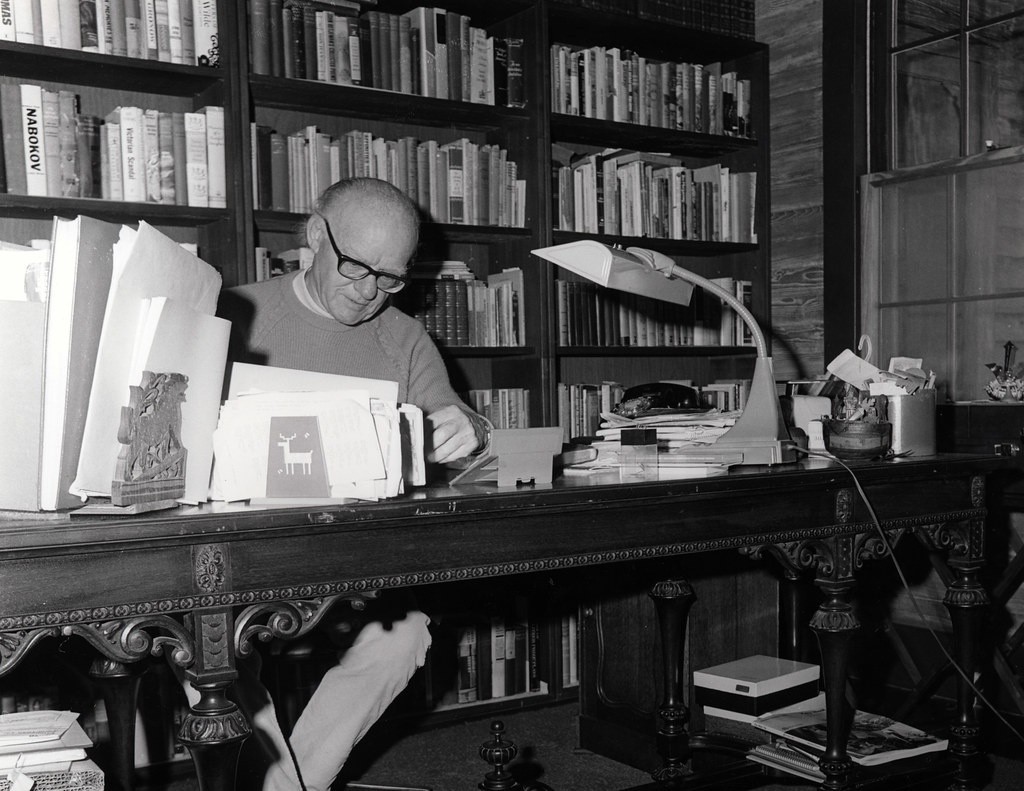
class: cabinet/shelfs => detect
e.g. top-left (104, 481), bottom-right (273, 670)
top-left (1, 0), bottom-right (774, 779)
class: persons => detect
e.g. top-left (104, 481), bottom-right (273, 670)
top-left (157, 175), bottom-right (494, 791)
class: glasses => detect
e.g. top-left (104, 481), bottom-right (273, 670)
top-left (324, 220), bottom-right (410, 295)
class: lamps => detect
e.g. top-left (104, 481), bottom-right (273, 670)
top-left (528, 242), bottom-right (799, 466)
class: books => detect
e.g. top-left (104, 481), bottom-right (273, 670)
top-left (0, 2), bottom-right (948, 788)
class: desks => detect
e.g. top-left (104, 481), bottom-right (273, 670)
top-left (0, 446), bottom-right (1023, 791)
